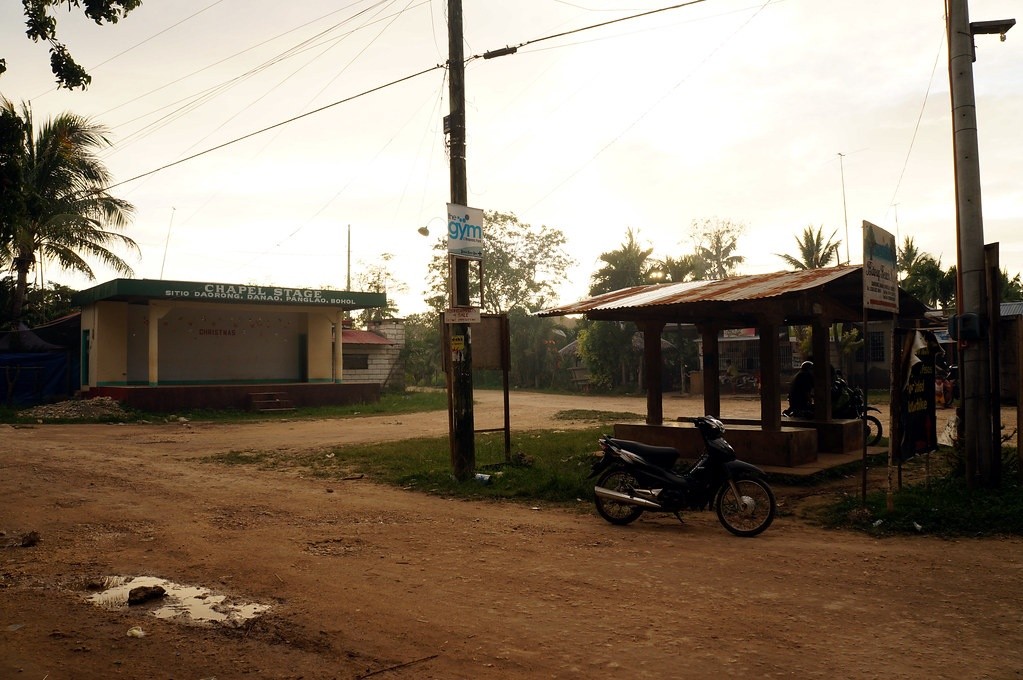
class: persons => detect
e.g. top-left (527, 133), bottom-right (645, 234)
top-left (935, 351), bottom-right (945, 375)
top-left (726, 359), bottom-right (737, 395)
top-left (789, 360), bottom-right (815, 418)
top-left (830, 364), bottom-right (850, 420)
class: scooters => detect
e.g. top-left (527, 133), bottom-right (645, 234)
top-left (780, 378), bottom-right (883, 446)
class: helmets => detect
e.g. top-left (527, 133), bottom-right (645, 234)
top-left (801, 361), bottom-right (816, 371)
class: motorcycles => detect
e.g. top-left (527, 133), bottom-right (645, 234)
top-left (586, 415), bottom-right (777, 537)
